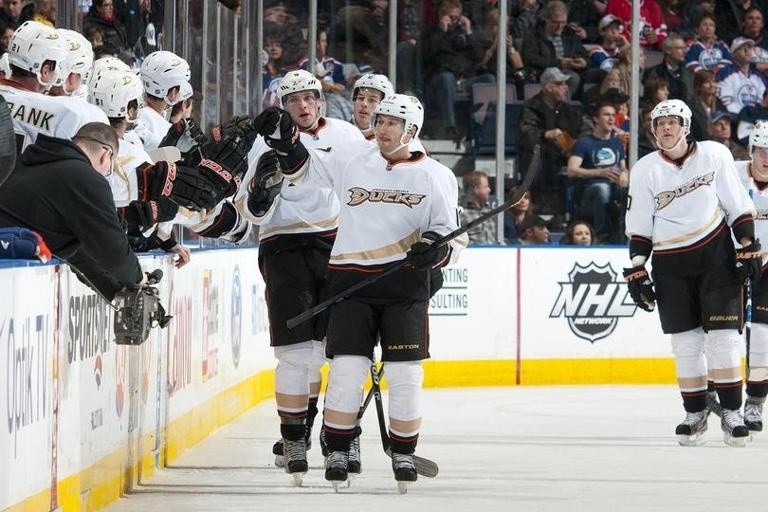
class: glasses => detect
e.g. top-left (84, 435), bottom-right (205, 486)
top-left (105, 158), bottom-right (112, 176)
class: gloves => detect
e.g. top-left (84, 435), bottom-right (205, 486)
top-left (130, 114), bottom-right (257, 233)
top-left (254, 107), bottom-right (310, 175)
top-left (623, 264), bottom-right (656, 312)
top-left (408, 232), bottom-right (450, 272)
top-left (246, 149), bottom-right (283, 217)
top-left (734, 238), bottom-right (762, 280)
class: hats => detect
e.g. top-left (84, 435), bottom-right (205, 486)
top-left (731, 37), bottom-right (755, 52)
top-left (600, 88), bottom-right (629, 104)
top-left (522, 215), bottom-right (549, 231)
top-left (540, 67), bottom-right (572, 86)
top-left (598, 14), bottom-right (622, 36)
top-left (710, 111), bottom-right (731, 123)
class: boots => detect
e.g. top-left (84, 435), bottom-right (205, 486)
top-left (273, 397), bottom-right (361, 481)
top-left (675, 379), bottom-right (768, 437)
top-left (389, 431), bottom-right (419, 481)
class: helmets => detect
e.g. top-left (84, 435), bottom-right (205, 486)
top-left (651, 99), bottom-right (692, 133)
top-left (8, 20), bottom-right (193, 118)
top-left (749, 120), bottom-right (768, 155)
top-left (372, 93), bottom-right (424, 138)
top-left (279, 70), bottom-right (322, 106)
top-left (351, 74), bottom-right (394, 102)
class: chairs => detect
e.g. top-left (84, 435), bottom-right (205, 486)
top-left (471, 78), bottom-right (517, 154)
top-left (524, 84), bottom-right (571, 103)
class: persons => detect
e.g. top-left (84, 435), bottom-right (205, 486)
top-left (623, 99), bottom-right (760, 440)
top-left (1, 0), bottom-right (257, 266)
top-left (707, 120), bottom-right (768, 430)
top-left (233, 68), bottom-right (366, 475)
top-left (262, 1), bottom-right (767, 246)
top-left (253, 92), bottom-right (461, 481)
top-left (271, 74), bottom-right (430, 474)
top-left (0, 121), bottom-right (147, 297)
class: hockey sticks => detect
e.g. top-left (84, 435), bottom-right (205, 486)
top-left (287, 145), bottom-right (540, 330)
top-left (371, 349), bottom-right (439, 478)
